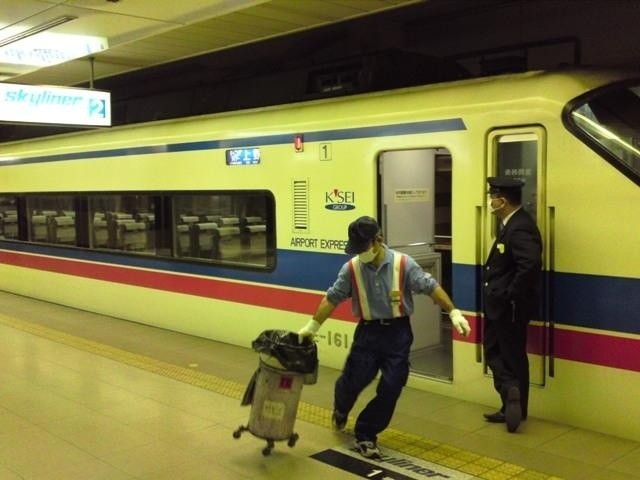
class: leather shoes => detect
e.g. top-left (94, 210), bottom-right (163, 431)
top-left (502, 380), bottom-right (522, 434)
top-left (483, 409), bottom-right (527, 423)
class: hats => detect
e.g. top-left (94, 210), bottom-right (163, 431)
top-left (344, 216), bottom-right (380, 255)
top-left (485, 176), bottom-right (526, 195)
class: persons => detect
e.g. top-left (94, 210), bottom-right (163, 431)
top-left (481, 175), bottom-right (542, 433)
top-left (296, 214), bottom-right (472, 459)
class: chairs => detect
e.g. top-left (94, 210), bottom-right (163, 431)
top-left (0, 207), bottom-right (267, 263)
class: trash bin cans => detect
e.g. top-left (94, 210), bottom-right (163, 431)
top-left (234, 330), bottom-right (314, 456)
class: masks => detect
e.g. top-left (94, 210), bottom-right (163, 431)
top-left (487, 197), bottom-right (505, 213)
top-left (359, 246), bottom-right (381, 264)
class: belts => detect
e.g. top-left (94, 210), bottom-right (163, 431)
top-left (364, 318), bottom-right (409, 325)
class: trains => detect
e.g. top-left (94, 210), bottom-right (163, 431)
top-left (0, 69), bottom-right (640, 445)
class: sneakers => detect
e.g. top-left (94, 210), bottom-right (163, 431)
top-left (332, 407), bottom-right (348, 431)
top-left (355, 438), bottom-right (381, 459)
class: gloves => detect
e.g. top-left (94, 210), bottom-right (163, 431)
top-left (448, 308), bottom-right (472, 338)
top-left (298, 318), bottom-right (322, 344)
